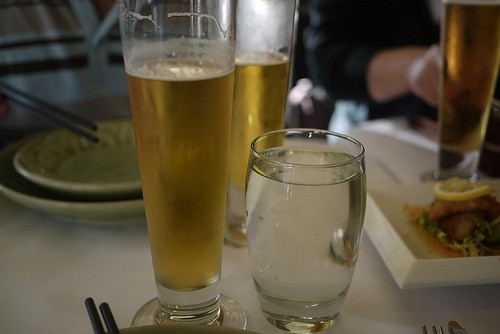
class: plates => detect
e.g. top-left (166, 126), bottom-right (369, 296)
top-left (14, 120), bottom-right (142, 200)
top-left (0, 131), bottom-right (147, 226)
top-left (364, 180), bottom-right (500, 289)
top-left (366, 116), bottom-right (439, 154)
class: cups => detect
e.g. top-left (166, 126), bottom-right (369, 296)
top-left (222, 0), bottom-right (299, 246)
top-left (244, 128), bottom-right (367, 334)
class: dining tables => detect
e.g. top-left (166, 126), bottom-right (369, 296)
top-left (0, 119), bottom-right (500, 334)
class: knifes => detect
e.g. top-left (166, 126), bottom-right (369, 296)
top-left (448, 320), bottom-right (466, 334)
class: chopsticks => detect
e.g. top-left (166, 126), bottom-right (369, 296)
top-left (0, 80), bottom-right (101, 143)
top-left (85, 296), bottom-right (121, 334)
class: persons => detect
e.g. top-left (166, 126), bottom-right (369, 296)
top-left (298, 0), bottom-right (500, 150)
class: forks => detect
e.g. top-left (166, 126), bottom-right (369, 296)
top-left (422, 325), bottom-right (444, 334)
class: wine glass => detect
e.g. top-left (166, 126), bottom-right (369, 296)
top-left (419, 0), bottom-right (500, 184)
top-left (118, 0), bottom-right (247, 329)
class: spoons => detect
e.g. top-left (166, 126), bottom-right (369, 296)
top-left (331, 228), bottom-right (358, 266)
